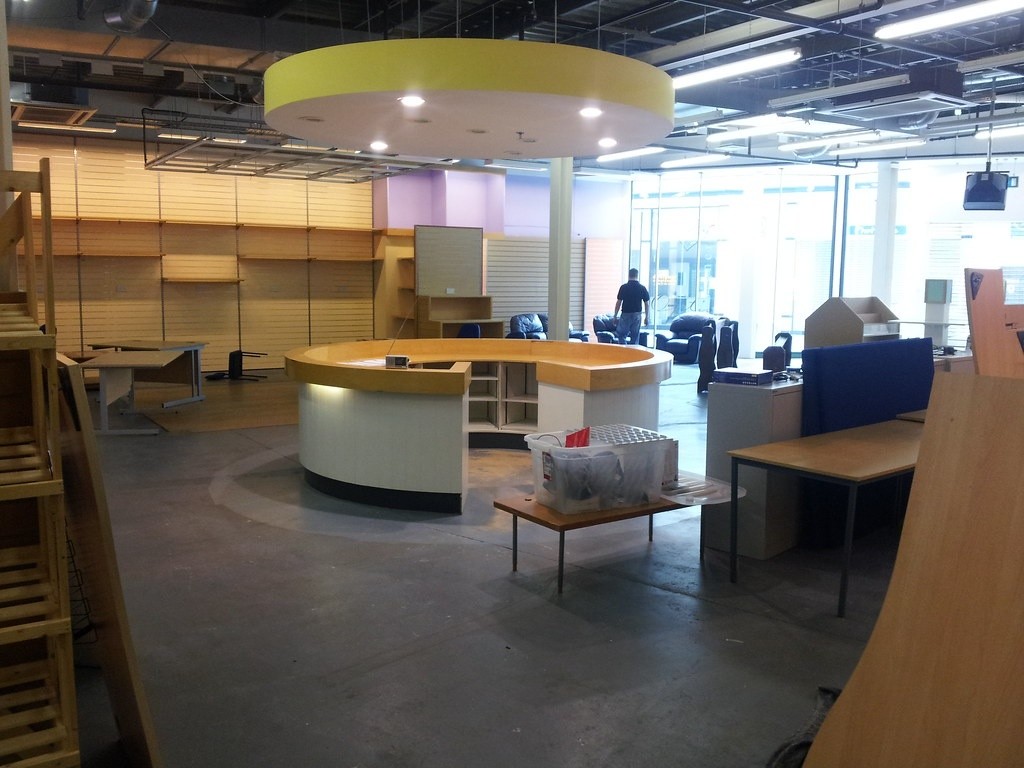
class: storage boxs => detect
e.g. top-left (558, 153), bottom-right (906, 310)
top-left (523, 431), bottom-right (673, 515)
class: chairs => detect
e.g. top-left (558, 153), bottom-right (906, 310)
top-left (204, 349), bottom-right (268, 382)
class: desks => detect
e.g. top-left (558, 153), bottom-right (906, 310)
top-left (494, 493), bottom-right (694, 595)
top-left (725, 421), bottom-right (924, 619)
top-left (59, 337), bottom-right (207, 436)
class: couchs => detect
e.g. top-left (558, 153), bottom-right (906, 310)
top-left (654, 310), bottom-right (717, 365)
top-left (505, 312), bottom-right (589, 343)
top-left (593, 313), bottom-right (650, 348)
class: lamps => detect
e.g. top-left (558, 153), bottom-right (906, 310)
top-left (873, 0), bottom-right (1024, 42)
top-left (671, 47), bottom-right (803, 90)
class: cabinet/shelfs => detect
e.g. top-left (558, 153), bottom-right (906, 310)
top-left (0, 157), bottom-right (81, 768)
top-left (417, 294), bottom-right (505, 338)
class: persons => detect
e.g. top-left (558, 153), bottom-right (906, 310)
top-left (612, 268), bottom-right (650, 345)
